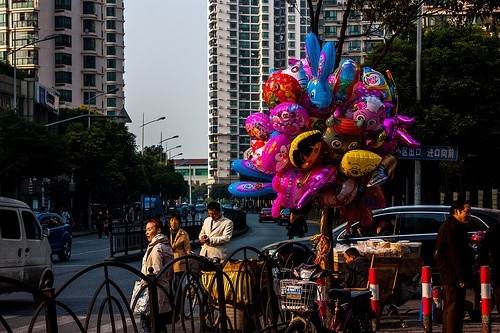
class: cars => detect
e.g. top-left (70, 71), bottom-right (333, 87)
top-left (277, 208), bottom-right (292, 226)
top-left (258, 205), bottom-right (500, 298)
top-left (35, 212), bottom-right (72, 262)
top-left (174, 201), bottom-right (242, 214)
top-left (258, 207), bottom-right (276, 223)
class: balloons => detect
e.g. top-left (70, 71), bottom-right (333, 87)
top-left (228, 32), bottom-right (421, 235)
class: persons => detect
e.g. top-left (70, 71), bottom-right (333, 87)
top-left (167, 212), bottom-right (192, 314)
top-left (476, 222), bottom-right (500, 317)
top-left (356, 218), bottom-right (389, 237)
top-left (333, 248), bottom-right (370, 313)
top-left (286, 213), bottom-right (308, 239)
top-left (119, 203), bottom-right (195, 238)
top-left (276, 242), bottom-right (305, 327)
top-left (198, 202), bottom-right (234, 320)
top-left (96, 209), bottom-right (112, 239)
top-left (139, 219), bottom-right (174, 333)
top-left (44, 205), bottom-right (72, 230)
top-left (436, 200), bottom-right (474, 333)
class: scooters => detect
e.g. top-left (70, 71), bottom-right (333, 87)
top-left (280, 269), bottom-right (375, 332)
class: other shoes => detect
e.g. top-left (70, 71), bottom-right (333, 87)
top-left (105, 236), bottom-right (108, 239)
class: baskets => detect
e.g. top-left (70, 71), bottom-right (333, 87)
top-left (279, 279), bottom-right (317, 313)
top-left (201, 304), bottom-right (246, 329)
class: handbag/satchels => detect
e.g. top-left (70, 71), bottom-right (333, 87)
top-left (130, 279), bottom-right (149, 314)
top-left (103, 222), bottom-right (108, 227)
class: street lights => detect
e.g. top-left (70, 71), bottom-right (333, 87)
top-left (85, 84), bottom-right (120, 229)
top-left (139, 113), bottom-right (165, 210)
top-left (159, 131), bottom-right (180, 213)
top-left (5, 24), bottom-right (60, 199)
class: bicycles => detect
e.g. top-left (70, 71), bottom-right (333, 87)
top-left (176, 251), bottom-right (222, 317)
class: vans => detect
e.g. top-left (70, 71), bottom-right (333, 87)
top-left (0, 197), bottom-right (55, 301)
